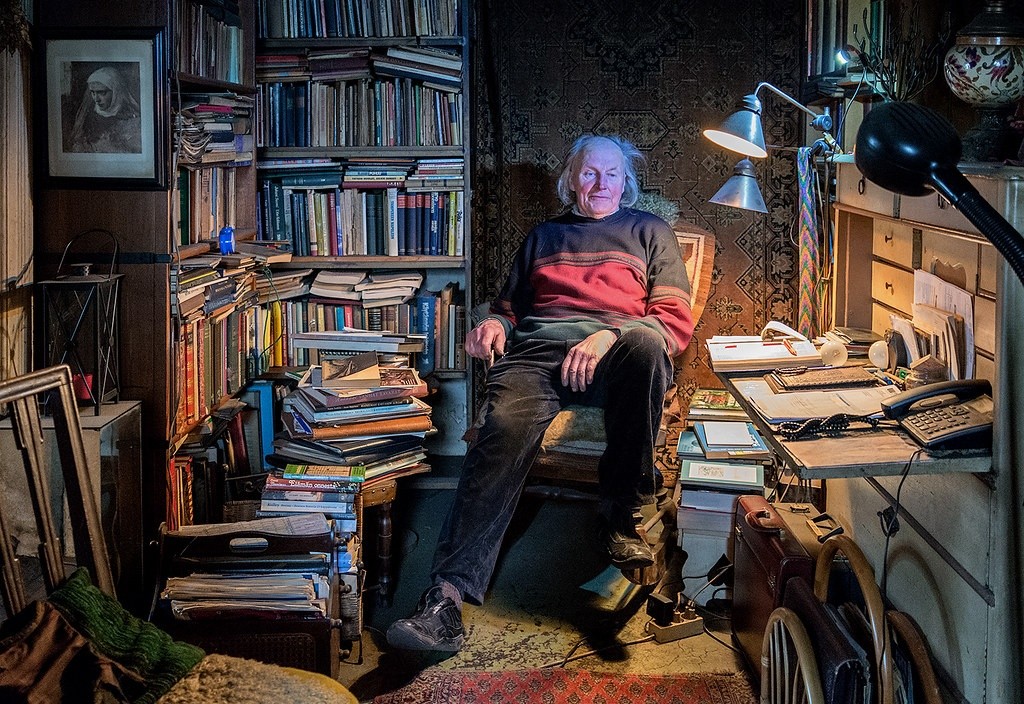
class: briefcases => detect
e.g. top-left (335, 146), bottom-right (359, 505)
top-left (727, 494), bottom-right (843, 672)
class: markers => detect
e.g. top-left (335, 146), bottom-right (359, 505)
top-left (783, 338), bottom-right (797, 356)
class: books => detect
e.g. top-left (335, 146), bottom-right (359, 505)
top-left (677, 389), bottom-right (771, 532)
top-left (893, 269), bottom-right (976, 381)
top-left (158, 0), bottom-right (463, 619)
top-left (704, 321), bottom-right (902, 424)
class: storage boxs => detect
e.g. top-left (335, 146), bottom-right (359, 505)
top-left (676, 503), bottom-right (733, 609)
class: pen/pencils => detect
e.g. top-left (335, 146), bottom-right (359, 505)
top-left (864, 367), bottom-right (905, 391)
top-left (763, 343), bottom-right (794, 346)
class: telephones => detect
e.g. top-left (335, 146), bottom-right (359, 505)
top-left (881, 379), bottom-right (993, 450)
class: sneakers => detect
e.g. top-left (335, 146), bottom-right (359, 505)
top-left (606, 530), bottom-right (654, 570)
top-left (385, 586), bottom-right (466, 651)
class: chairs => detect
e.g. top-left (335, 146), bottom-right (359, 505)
top-left (483, 195), bottom-right (716, 614)
top-left (0, 364), bottom-right (361, 703)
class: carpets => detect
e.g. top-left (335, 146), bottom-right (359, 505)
top-left (372, 667), bottom-right (758, 704)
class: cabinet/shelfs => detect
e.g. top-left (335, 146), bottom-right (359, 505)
top-left (34, 0), bottom-right (476, 647)
top-left (699, 162), bottom-right (1024, 704)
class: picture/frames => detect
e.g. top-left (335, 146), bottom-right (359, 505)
top-left (36, 24), bottom-right (170, 194)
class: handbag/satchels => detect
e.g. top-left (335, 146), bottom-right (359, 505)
top-left (761, 534), bottom-right (943, 704)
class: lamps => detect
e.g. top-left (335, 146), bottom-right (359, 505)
top-left (702, 81), bottom-right (832, 159)
top-left (855, 101), bottom-right (1024, 289)
top-left (708, 146), bottom-right (802, 214)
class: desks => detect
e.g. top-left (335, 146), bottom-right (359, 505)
top-left (363, 479), bottom-right (399, 610)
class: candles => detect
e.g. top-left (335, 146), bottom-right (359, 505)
top-left (73, 374), bottom-right (92, 399)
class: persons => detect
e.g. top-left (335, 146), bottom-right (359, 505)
top-left (385, 132), bottom-right (694, 651)
